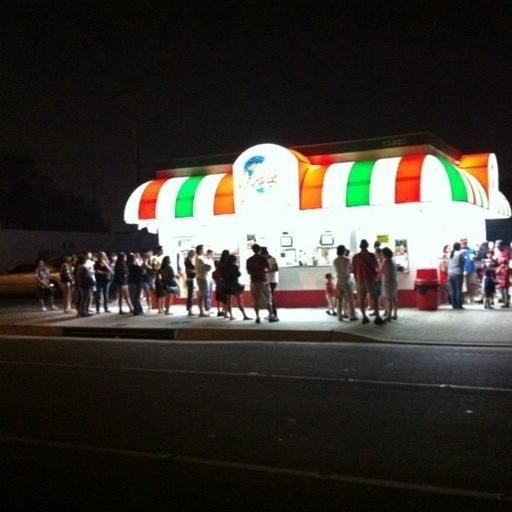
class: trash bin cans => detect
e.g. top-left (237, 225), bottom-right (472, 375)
top-left (414, 268), bottom-right (441, 310)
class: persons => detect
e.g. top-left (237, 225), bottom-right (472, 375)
top-left (34, 242), bottom-right (279, 323)
top-left (439, 237), bottom-right (512, 309)
top-left (324, 239), bottom-right (399, 326)
